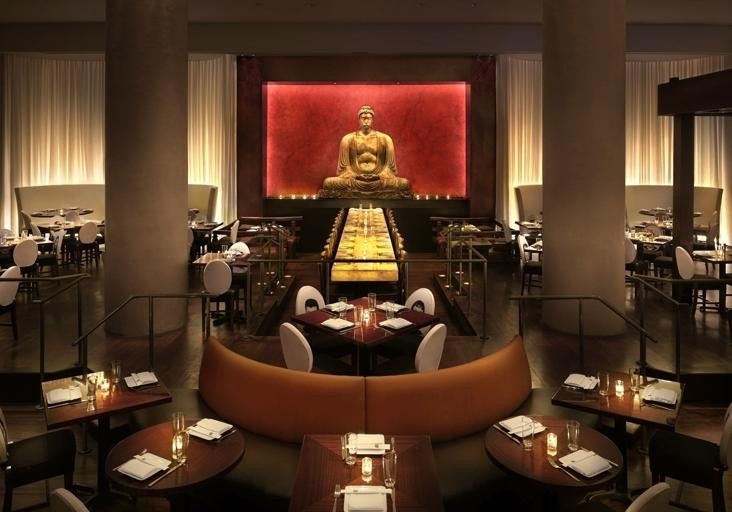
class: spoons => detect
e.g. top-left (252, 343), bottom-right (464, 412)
top-left (389, 437), bottom-right (396, 455)
top-left (112, 448), bottom-right (147, 473)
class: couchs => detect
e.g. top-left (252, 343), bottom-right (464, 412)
top-left (199, 334), bottom-right (533, 443)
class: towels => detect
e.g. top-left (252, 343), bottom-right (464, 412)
top-left (326, 298), bottom-right (355, 313)
top-left (565, 371), bottom-right (597, 391)
top-left (190, 414), bottom-right (233, 445)
top-left (560, 446), bottom-right (608, 479)
top-left (353, 430), bottom-right (385, 455)
top-left (115, 452), bottom-right (165, 482)
top-left (321, 314), bottom-right (355, 334)
top-left (377, 314), bottom-right (411, 332)
top-left (377, 297), bottom-right (403, 314)
top-left (46, 384), bottom-right (81, 408)
top-left (502, 412), bottom-right (539, 441)
top-left (642, 377), bottom-right (677, 409)
top-left (348, 483), bottom-right (388, 511)
top-left (123, 368), bottom-right (160, 389)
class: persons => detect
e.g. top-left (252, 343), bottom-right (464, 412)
top-left (322, 106), bottom-right (410, 191)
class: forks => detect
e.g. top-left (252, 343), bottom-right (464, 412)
top-left (216, 429), bottom-right (236, 443)
top-left (547, 456), bottom-right (580, 482)
top-left (331, 483), bottom-right (340, 512)
top-left (371, 322), bottom-right (393, 334)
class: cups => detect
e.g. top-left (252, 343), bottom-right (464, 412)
top-left (636, 234), bottom-right (640, 239)
top-left (23, 231), bottom-right (28, 239)
top-left (662, 216), bottom-right (667, 225)
top-left (226, 254), bottom-right (231, 263)
top-left (110, 360), bottom-right (119, 383)
top-left (625, 228), bottom-right (629, 239)
top-left (366, 292), bottom-right (376, 311)
top-left (86, 376), bottom-right (98, 404)
top-left (45, 234), bottom-right (50, 243)
top-left (716, 246), bottom-right (723, 258)
top-left (21, 233), bottom-right (27, 241)
top-left (59, 209), bottom-right (65, 216)
top-left (649, 238), bottom-right (653, 243)
top-left (231, 254), bottom-right (236, 263)
top-left (337, 296), bottom-right (347, 318)
top-left (381, 453), bottom-right (396, 487)
top-left (521, 417), bottom-right (534, 451)
top-left (384, 300), bottom-right (395, 318)
top-left (222, 245), bottom-right (228, 257)
top-left (352, 305), bottom-right (362, 327)
top-left (641, 237), bottom-right (646, 242)
top-left (566, 419), bottom-right (579, 450)
top-left (630, 230), bottom-right (635, 240)
top-left (597, 371), bottom-right (609, 395)
top-left (343, 432), bottom-right (357, 465)
top-left (60, 224), bottom-right (65, 232)
top-left (628, 367), bottom-right (640, 391)
top-left (654, 218), bottom-right (659, 227)
top-left (170, 411), bottom-right (184, 432)
top-left (0, 233), bottom-right (8, 248)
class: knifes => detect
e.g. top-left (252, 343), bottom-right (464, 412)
top-left (339, 435), bottom-right (346, 459)
top-left (580, 445), bottom-right (618, 466)
top-left (390, 486), bottom-right (396, 512)
top-left (146, 462), bottom-right (184, 488)
top-left (45, 397), bottom-right (85, 407)
top-left (492, 424), bottom-right (521, 445)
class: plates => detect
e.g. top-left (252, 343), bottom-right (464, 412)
top-left (498, 415), bottom-right (545, 438)
top-left (343, 485), bottom-right (387, 512)
top-left (642, 384), bottom-right (678, 411)
top-left (325, 301), bottom-right (354, 312)
top-left (123, 371), bottom-right (158, 388)
top-left (563, 373), bottom-right (599, 390)
top-left (185, 418), bottom-right (233, 441)
top-left (349, 433), bottom-right (385, 455)
top-left (379, 317), bottom-right (412, 330)
top-left (46, 387), bottom-right (82, 404)
top-left (321, 316), bottom-right (354, 330)
top-left (556, 448), bottom-right (612, 478)
top-left (117, 452), bottom-right (171, 481)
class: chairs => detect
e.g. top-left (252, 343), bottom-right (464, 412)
top-left (20, 209), bottom-right (36, 234)
top-left (519, 248), bottom-right (543, 295)
top-left (225, 242), bottom-right (250, 314)
top-left (0, 265), bottom-right (22, 341)
top-left (1, 239), bottom-right (40, 298)
top-left (30, 221), bottom-right (57, 272)
top-left (674, 246), bottom-right (724, 317)
top-left (576, 481), bottom-right (671, 511)
top-left (199, 260), bottom-right (237, 337)
top-left (279, 322), bottom-right (355, 378)
top-left (75, 222), bottom-right (101, 272)
top-left (517, 232), bottom-right (540, 279)
top-left (48, 486), bottom-right (174, 511)
top-left (373, 324), bottom-right (448, 377)
top-left (380, 287), bottom-right (436, 356)
top-left (0, 408), bottom-right (78, 512)
top-left (648, 399), bottom-right (732, 512)
top-left (293, 286), bottom-right (357, 359)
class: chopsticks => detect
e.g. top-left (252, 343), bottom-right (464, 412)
top-left (132, 454), bottom-right (169, 471)
top-left (346, 444), bottom-right (390, 449)
top-left (375, 301), bottom-right (406, 312)
top-left (340, 488), bottom-right (392, 494)
top-left (562, 450), bottom-right (594, 467)
top-left (130, 372), bottom-right (143, 386)
top-left (188, 423), bottom-right (221, 439)
top-left (508, 422), bottom-right (540, 435)
